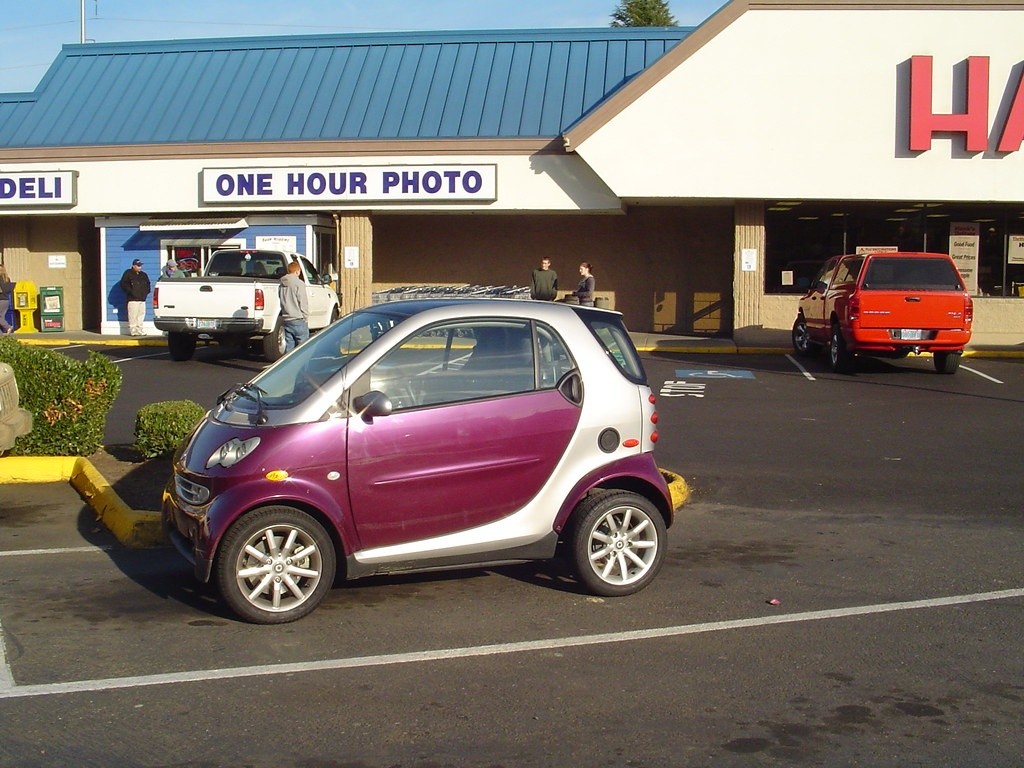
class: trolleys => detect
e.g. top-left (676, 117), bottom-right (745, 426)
top-left (371, 284), bottom-right (535, 338)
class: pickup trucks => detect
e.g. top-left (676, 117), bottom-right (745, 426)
top-left (153, 247), bottom-right (345, 362)
top-left (792, 253), bottom-right (974, 374)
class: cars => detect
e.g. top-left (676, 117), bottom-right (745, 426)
top-left (162, 297), bottom-right (690, 624)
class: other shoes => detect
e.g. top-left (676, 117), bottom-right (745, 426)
top-left (138, 331), bottom-right (147, 336)
top-left (129, 332), bottom-right (140, 336)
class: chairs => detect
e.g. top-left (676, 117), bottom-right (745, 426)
top-left (425, 331), bottom-right (531, 405)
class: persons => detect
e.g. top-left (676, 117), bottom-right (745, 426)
top-left (120, 259), bottom-right (151, 336)
top-left (0, 264), bottom-right (14, 334)
top-left (530, 257), bottom-right (559, 302)
top-left (278, 262), bottom-right (310, 354)
top-left (162, 259), bottom-right (185, 279)
top-left (572, 262), bottom-right (596, 307)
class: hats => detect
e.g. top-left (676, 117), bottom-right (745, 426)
top-left (133, 259), bottom-right (144, 266)
top-left (167, 259), bottom-right (178, 266)
